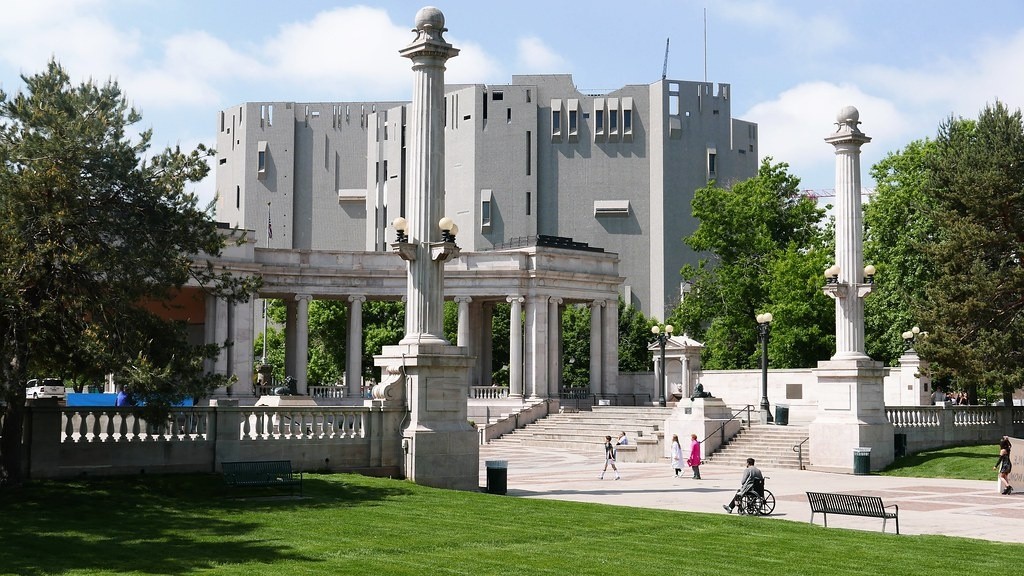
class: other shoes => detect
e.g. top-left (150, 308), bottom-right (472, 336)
top-left (724, 505), bottom-right (732, 514)
top-left (693, 477), bottom-right (701, 479)
top-left (614, 477), bottom-right (620, 480)
top-left (678, 469), bottom-right (684, 477)
top-left (672, 475), bottom-right (678, 478)
top-left (1002, 486), bottom-right (1013, 495)
top-left (596, 476), bottom-right (603, 480)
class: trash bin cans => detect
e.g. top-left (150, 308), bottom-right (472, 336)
top-left (854, 446), bottom-right (871, 473)
top-left (486, 460), bottom-right (508, 494)
top-left (775, 403), bottom-right (788, 425)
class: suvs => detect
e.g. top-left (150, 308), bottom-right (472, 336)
top-left (26, 377), bottom-right (65, 401)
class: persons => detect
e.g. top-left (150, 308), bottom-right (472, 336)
top-left (722, 458), bottom-right (762, 514)
top-left (948, 391), bottom-right (968, 405)
top-left (931, 386), bottom-right (943, 401)
top-left (691, 434), bottom-right (701, 479)
top-left (257, 379), bottom-right (262, 385)
top-left (596, 436), bottom-right (620, 480)
top-left (993, 436), bottom-right (1013, 495)
top-left (614, 432), bottom-right (628, 456)
top-left (671, 434), bottom-right (684, 478)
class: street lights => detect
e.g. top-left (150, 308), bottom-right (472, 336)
top-left (394, 6), bottom-right (462, 342)
top-left (651, 324), bottom-right (673, 406)
top-left (821, 106), bottom-right (876, 360)
top-left (755, 311), bottom-right (773, 422)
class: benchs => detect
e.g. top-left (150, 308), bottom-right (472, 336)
top-left (221, 459), bottom-right (303, 500)
top-left (806, 491), bottom-right (900, 535)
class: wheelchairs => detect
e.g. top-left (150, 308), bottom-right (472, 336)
top-left (734, 477), bottom-right (776, 516)
